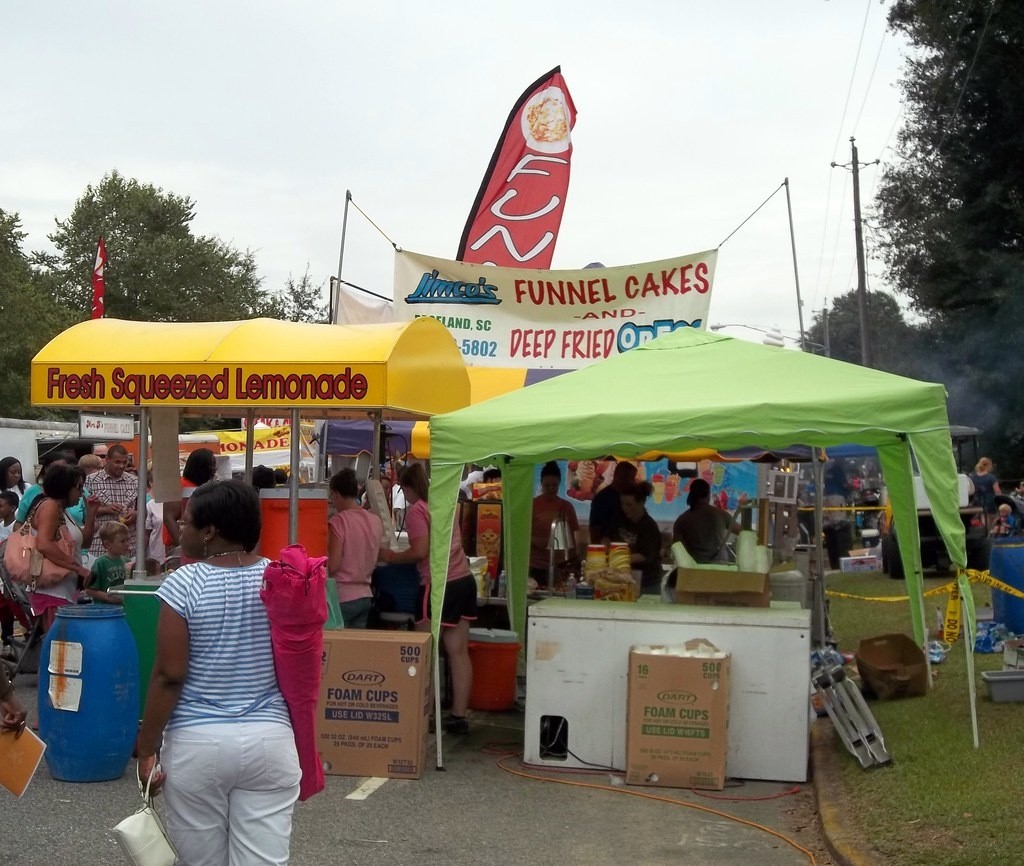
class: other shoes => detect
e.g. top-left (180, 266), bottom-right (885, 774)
top-left (442, 715), bottom-right (469, 735)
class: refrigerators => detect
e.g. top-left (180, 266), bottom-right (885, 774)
top-left (523, 596), bottom-right (813, 783)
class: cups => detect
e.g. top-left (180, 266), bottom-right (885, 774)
top-left (584, 542), bottom-right (630, 582)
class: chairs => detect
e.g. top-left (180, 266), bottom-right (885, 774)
top-left (0, 556), bottom-right (52, 689)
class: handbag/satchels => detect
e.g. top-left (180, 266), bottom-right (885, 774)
top-left (1, 500), bottom-right (75, 586)
top-left (110, 753), bottom-right (180, 866)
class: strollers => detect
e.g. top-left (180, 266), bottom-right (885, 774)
top-left (987, 493), bottom-right (1024, 540)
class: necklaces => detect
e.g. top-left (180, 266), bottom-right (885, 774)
top-left (208, 550), bottom-right (248, 564)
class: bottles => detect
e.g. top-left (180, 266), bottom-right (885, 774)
top-left (566, 573), bottom-right (577, 599)
top-left (498, 570), bottom-right (506, 598)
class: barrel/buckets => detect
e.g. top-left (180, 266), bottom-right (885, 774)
top-left (468, 627), bottom-right (521, 711)
top-left (37, 606), bottom-right (140, 784)
top-left (258, 488), bottom-right (329, 563)
top-left (989, 537), bottom-right (1024, 635)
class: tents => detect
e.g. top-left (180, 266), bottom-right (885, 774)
top-left (31, 317), bottom-right (472, 421)
top-left (316, 326), bottom-right (980, 770)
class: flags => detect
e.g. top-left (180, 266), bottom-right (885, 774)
top-left (90, 239), bottom-right (107, 319)
top-left (462, 70), bottom-right (579, 270)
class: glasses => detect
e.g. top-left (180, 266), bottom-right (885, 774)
top-left (74, 484), bottom-right (84, 492)
top-left (177, 519), bottom-right (192, 529)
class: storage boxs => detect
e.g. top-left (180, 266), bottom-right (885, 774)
top-left (977, 667), bottom-right (1023, 706)
top-left (855, 630), bottom-right (933, 703)
top-left (840, 555), bottom-right (884, 575)
top-left (320, 631), bottom-right (435, 780)
top-left (624, 636), bottom-right (731, 795)
top-left (673, 562), bottom-right (771, 607)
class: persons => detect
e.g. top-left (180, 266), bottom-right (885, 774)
top-left (0, 446), bottom-right (219, 740)
top-left (327, 455), bottom-right (1024, 732)
top-left (137, 478), bottom-right (302, 866)
